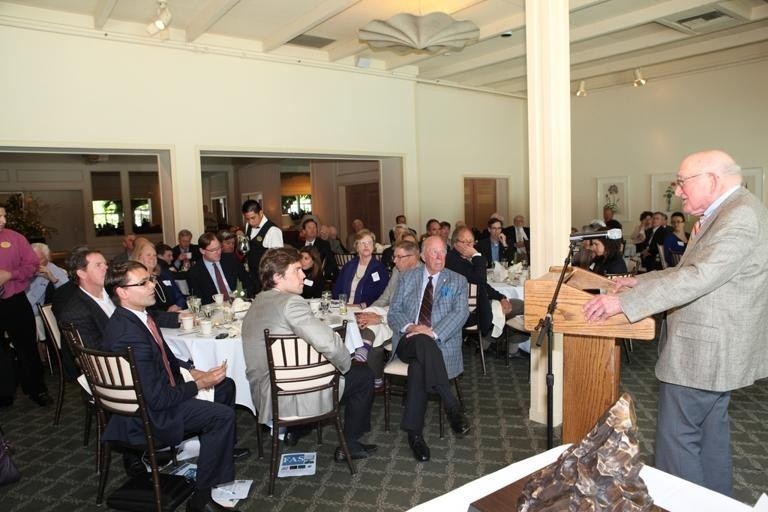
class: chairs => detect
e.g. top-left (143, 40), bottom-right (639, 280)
top-left (68, 331), bottom-right (208, 510)
top-left (573, 237), bottom-right (689, 363)
top-left (254, 319), bottom-right (357, 498)
top-left (380, 324), bottom-right (467, 441)
top-left (3, 236), bottom-right (524, 377)
top-left (35, 301), bottom-right (91, 431)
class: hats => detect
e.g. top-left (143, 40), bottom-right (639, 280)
top-left (217, 228), bottom-right (238, 241)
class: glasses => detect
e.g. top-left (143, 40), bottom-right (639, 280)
top-left (391, 255), bottom-right (414, 261)
top-left (678, 173), bottom-right (706, 189)
top-left (671, 221), bottom-right (683, 225)
top-left (358, 240), bottom-right (374, 245)
top-left (206, 248), bottom-right (222, 253)
top-left (489, 226), bottom-right (502, 229)
top-left (427, 248), bottom-right (447, 255)
top-left (457, 238), bottom-right (478, 245)
top-left (117, 274), bottom-right (153, 288)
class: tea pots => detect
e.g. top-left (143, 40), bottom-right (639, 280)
top-left (235, 236), bottom-right (252, 256)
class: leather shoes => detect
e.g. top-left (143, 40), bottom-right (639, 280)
top-left (29, 391), bottom-right (54, 408)
top-left (476, 345), bottom-right (507, 359)
top-left (233, 447), bottom-right (250, 460)
top-left (508, 349), bottom-right (529, 361)
top-left (448, 409), bottom-right (471, 434)
top-left (408, 433), bottom-right (430, 461)
top-left (334, 443), bottom-right (379, 464)
top-left (0, 394), bottom-right (17, 409)
top-left (285, 430), bottom-right (304, 446)
top-left (186, 497), bottom-right (240, 512)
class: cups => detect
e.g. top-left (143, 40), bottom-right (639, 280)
top-left (308, 290), bottom-right (347, 316)
top-left (483, 258), bottom-right (530, 288)
top-left (178, 294), bottom-right (255, 339)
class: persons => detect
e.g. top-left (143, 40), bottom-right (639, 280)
top-left (28, 226), bottom-right (250, 354)
top-left (239, 200), bottom-right (283, 299)
top-left (103, 261), bottom-right (237, 492)
top-left (583, 150), bottom-right (768, 496)
top-left (242, 247), bottom-right (377, 460)
top-left (0, 205), bottom-right (54, 406)
top-left (386, 236), bottom-right (472, 462)
top-left (573, 205), bottom-right (691, 278)
top-left (292, 215), bottom-right (530, 355)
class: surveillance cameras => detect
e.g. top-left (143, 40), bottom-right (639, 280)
top-left (146, 9), bottom-right (172, 37)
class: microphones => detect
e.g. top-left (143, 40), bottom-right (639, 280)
top-left (569, 228), bottom-right (623, 240)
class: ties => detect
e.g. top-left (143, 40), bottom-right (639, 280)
top-left (418, 275), bottom-right (434, 330)
top-left (184, 248), bottom-right (187, 265)
top-left (212, 263), bottom-right (230, 302)
top-left (517, 228), bottom-right (522, 242)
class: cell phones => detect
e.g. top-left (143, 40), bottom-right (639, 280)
top-left (215, 333), bottom-right (228, 339)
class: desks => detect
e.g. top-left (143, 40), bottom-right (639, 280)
top-left (410, 441), bottom-right (768, 512)
